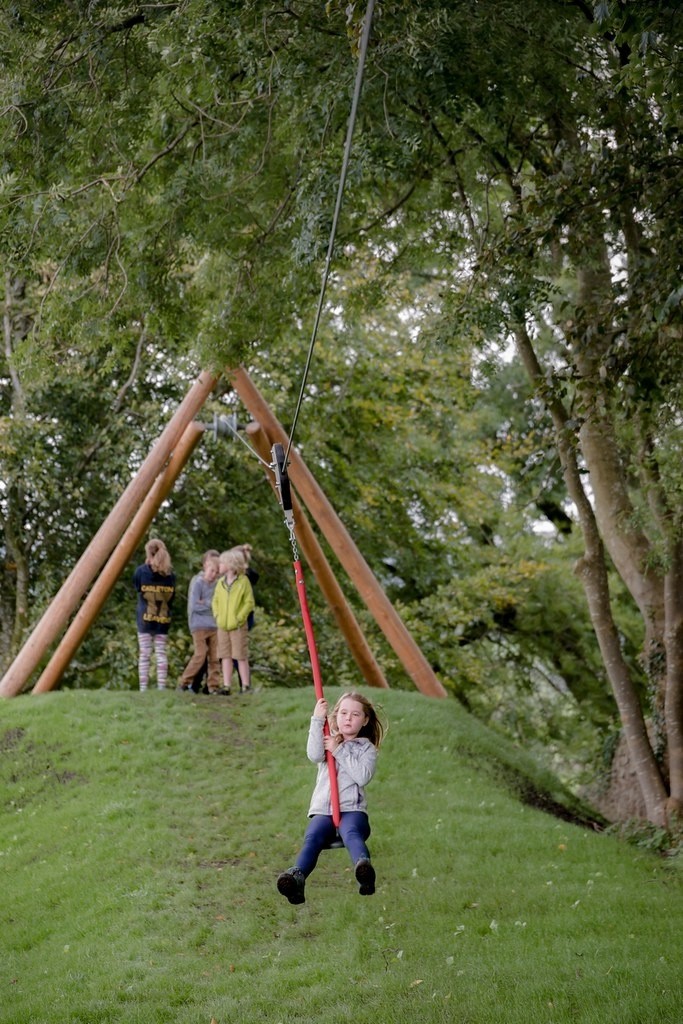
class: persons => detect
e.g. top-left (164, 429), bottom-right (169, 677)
top-left (133, 539), bottom-right (176, 691)
top-left (276, 692), bottom-right (382, 906)
top-left (177, 544), bottom-right (254, 696)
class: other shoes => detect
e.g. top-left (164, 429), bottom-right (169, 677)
top-left (213, 686), bottom-right (230, 695)
top-left (277, 867), bottom-right (305, 905)
top-left (176, 686), bottom-right (190, 692)
top-left (355, 857), bottom-right (376, 895)
